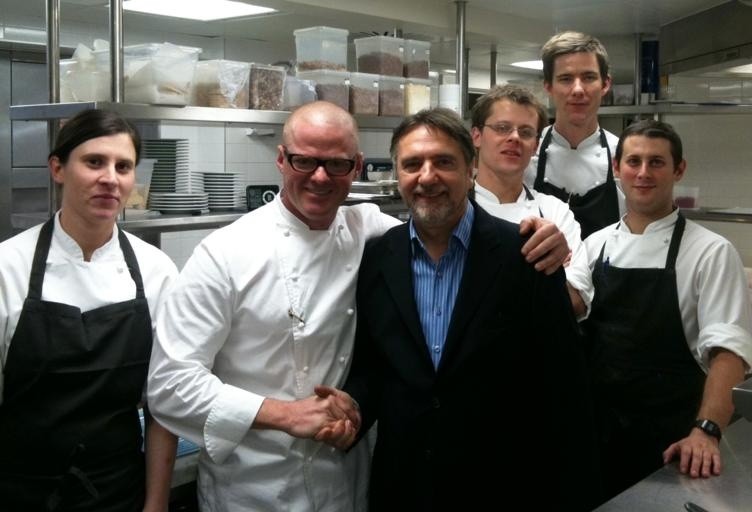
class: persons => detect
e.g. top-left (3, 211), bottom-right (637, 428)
top-left (146, 101), bottom-right (573, 510)
top-left (584, 119), bottom-right (751, 512)
top-left (313, 105), bottom-right (582, 510)
top-left (0, 109), bottom-right (178, 511)
top-left (520, 31), bottom-right (628, 242)
top-left (467, 86), bottom-right (596, 324)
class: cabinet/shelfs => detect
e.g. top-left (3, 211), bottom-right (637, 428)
top-left (11, 1), bottom-right (467, 249)
top-left (595, 105), bottom-right (752, 224)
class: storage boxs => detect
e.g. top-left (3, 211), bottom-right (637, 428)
top-left (293, 25), bottom-right (349, 70)
top-left (284, 77), bottom-right (317, 112)
top-left (354, 36), bottom-right (405, 78)
top-left (199, 60), bottom-right (249, 109)
top-left (349, 72), bottom-right (378, 116)
top-left (59, 44), bottom-right (202, 104)
top-left (379, 75), bottom-right (407, 116)
top-left (404, 38), bottom-right (433, 80)
top-left (248, 63), bottom-right (283, 111)
top-left (407, 77), bottom-right (433, 116)
top-left (295, 71), bottom-right (350, 113)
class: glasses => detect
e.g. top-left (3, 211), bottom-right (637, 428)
top-left (477, 121), bottom-right (542, 140)
top-left (282, 142), bottom-right (357, 178)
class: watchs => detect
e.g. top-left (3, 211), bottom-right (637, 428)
top-left (693, 417), bottom-right (722, 443)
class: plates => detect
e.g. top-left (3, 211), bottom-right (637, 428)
top-left (148, 192), bottom-right (208, 212)
top-left (190, 170), bottom-right (247, 210)
top-left (139, 137), bottom-right (189, 192)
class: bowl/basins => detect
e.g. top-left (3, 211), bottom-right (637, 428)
top-left (673, 185), bottom-right (700, 208)
